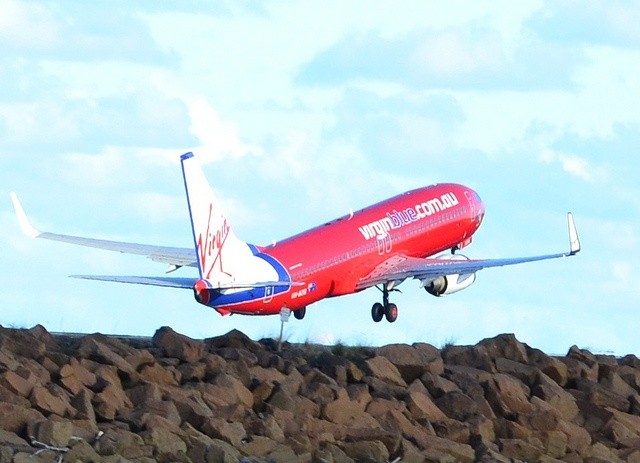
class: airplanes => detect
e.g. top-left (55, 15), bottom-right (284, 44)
top-left (35, 152), bottom-right (580, 322)
